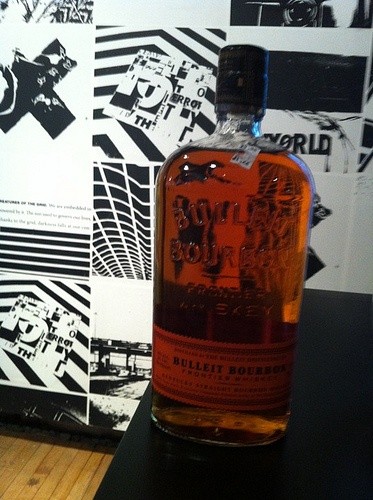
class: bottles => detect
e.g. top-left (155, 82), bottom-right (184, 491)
top-left (148, 43), bottom-right (318, 448)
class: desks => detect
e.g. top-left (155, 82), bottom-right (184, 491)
top-left (89, 285), bottom-right (373, 498)
